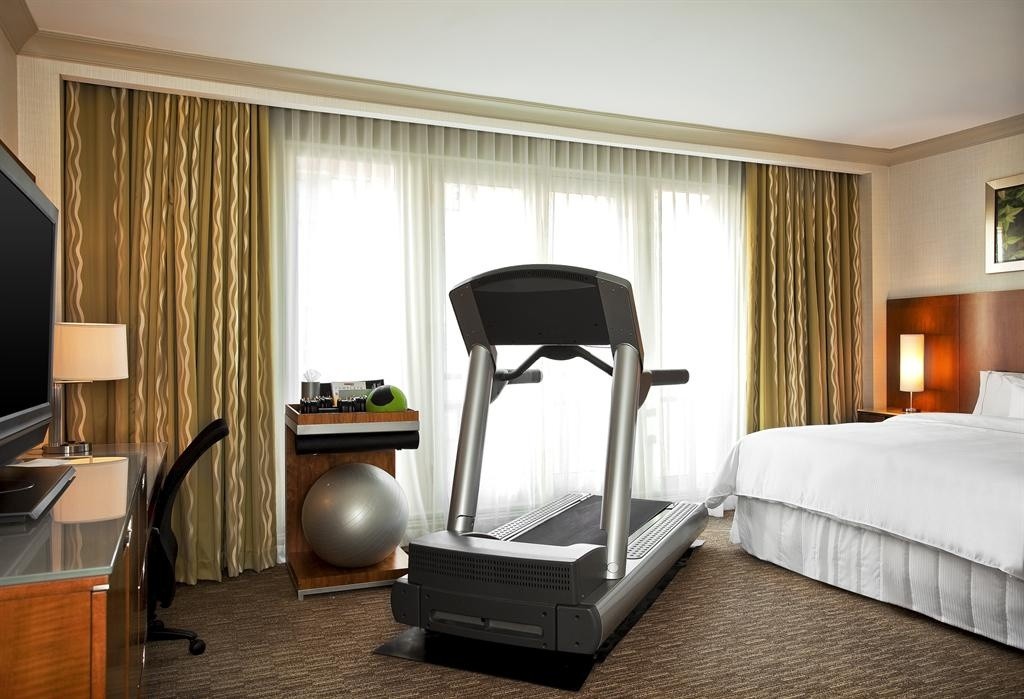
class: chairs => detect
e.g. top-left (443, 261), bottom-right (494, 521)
top-left (145, 416), bottom-right (231, 654)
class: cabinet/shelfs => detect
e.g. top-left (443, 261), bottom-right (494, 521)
top-left (0, 442), bottom-right (147, 699)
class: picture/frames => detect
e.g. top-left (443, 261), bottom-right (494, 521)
top-left (983, 173), bottom-right (1024, 275)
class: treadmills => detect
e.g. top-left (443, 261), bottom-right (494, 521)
top-left (390, 261), bottom-right (709, 655)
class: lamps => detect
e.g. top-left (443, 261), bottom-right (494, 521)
top-left (42, 323), bottom-right (128, 454)
top-left (897, 333), bottom-right (924, 413)
top-left (49, 453), bottom-right (129, 524)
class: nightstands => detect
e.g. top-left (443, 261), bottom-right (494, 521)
top-left (857, 407), bottom-right (909, 423)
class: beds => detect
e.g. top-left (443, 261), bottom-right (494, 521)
top-left (705, 288), bottom-right (1024, 652)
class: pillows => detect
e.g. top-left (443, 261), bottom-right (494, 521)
top-left (971, 370), bottom-right (1024, 419)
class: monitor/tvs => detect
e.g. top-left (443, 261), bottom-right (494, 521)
top-left (1, 144), bottom-right (77, 525)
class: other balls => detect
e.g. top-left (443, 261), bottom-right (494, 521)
top-left (366, 384), bottom-right (407, 410)
top-left (301, 463), bottom-right (409, 566)
